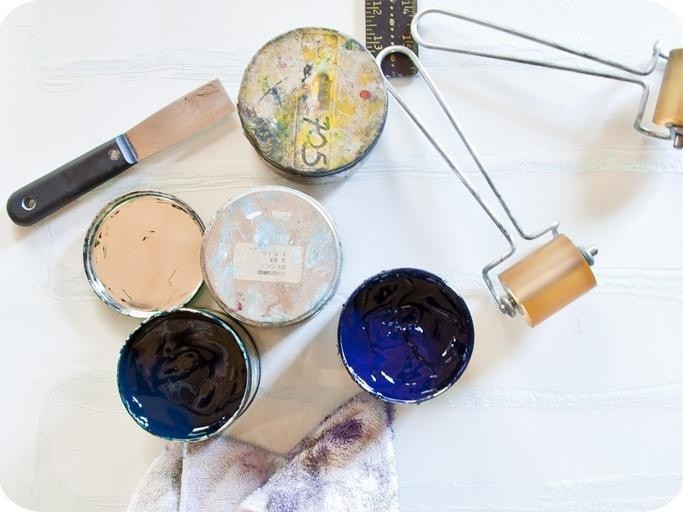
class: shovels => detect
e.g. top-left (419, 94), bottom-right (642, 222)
top-left (6, 77), bottom-right (237, 227)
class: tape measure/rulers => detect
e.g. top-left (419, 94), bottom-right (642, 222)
top-left (363, 0), bottom-right (419, 78)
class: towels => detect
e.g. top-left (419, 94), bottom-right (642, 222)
top-left (126, 393), bottom-right (400, 512)
